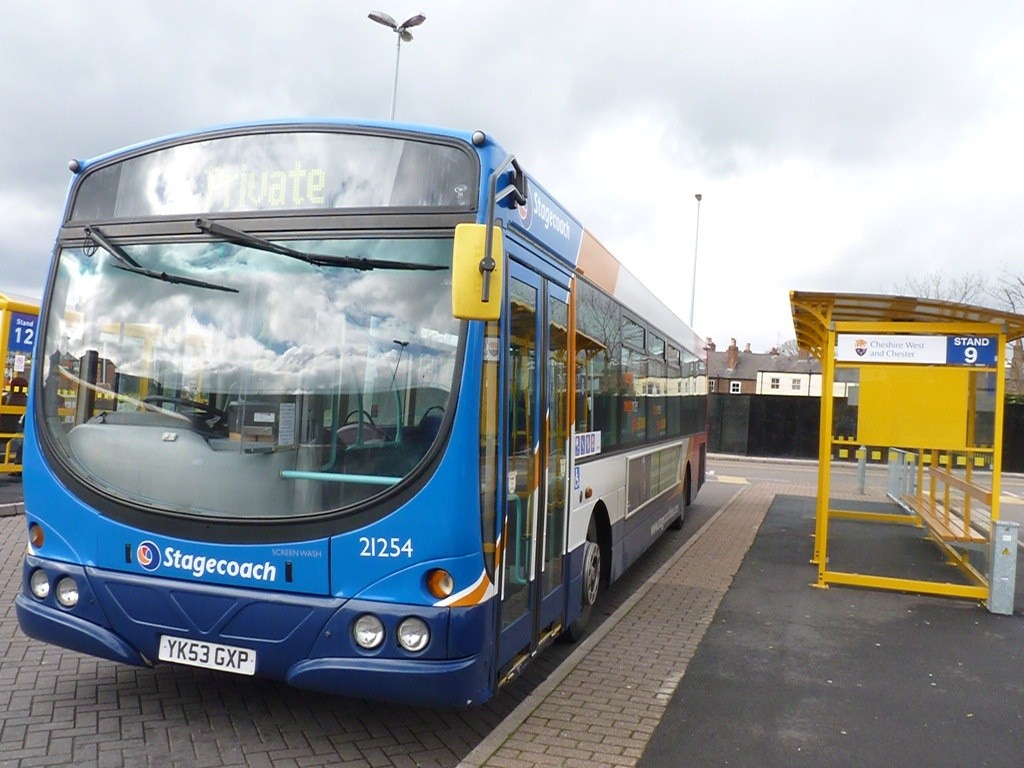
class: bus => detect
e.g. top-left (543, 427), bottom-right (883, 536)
top-left (16, 121), bottom-right (709, 704)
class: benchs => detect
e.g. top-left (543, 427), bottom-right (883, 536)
top-left (933, 491), bottom-right (990, 540)
top-left (898, 466), bottom-right (993, 553)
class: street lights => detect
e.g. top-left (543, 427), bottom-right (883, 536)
top-left (691, 193), bottom-right (702, 329)
top-left (369, 12), bottom-right (426, 121)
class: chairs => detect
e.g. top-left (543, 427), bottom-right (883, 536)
top-left (413, 406), bottom-right (447, 444)
top-left (343, 409), bottom-right (376, 427)
top-left (222, 380), bottom-right (289, 416)
